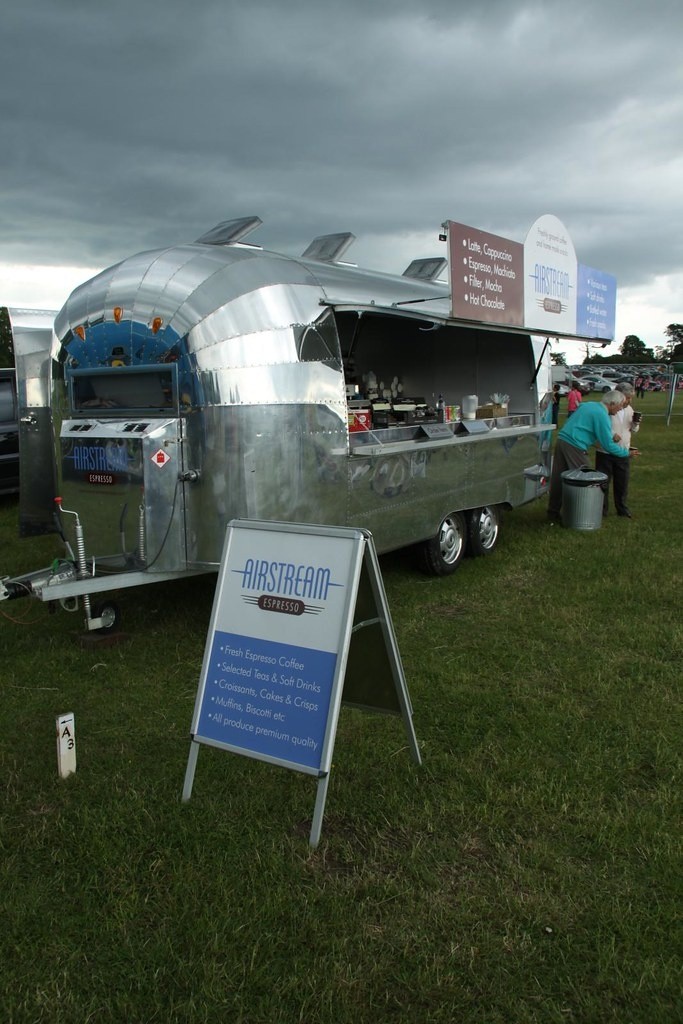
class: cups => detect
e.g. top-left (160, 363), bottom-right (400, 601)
top-left (629, 447), bottom-right (638, 450)
top-left (634, 412), bottom-right (641, 422)
top-left (493, 403), bottom-right (508, 418)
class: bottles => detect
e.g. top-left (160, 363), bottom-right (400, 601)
top-left (436, 394), bottom-right (446, 423)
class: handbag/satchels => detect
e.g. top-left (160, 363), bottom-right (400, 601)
top-left (574, 400), bottom-right (580, 407)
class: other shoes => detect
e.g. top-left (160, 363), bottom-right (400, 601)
top-left (555, 516), bottom-right (561, 522)
top-left (623, 510), bottom-right (631, 517)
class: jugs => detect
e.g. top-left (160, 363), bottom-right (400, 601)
top-left (462, 394), bottom-right (478, 420)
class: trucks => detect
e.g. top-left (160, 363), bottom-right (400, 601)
top-left (2, 214), bottom-right (559, 634)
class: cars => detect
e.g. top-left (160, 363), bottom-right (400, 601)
top-left (550, 364), bottom-right (683, 398)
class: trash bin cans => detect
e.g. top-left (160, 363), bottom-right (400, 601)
top-left (560, 469), bottom-right (608, 531)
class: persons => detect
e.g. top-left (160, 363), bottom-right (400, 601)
top-left (552, 384), bottom-right (560, 429)
top-left (547, 390), bottom-right (642, 522)
top-left (594, 381), bottom-right (642, 518)
top-left (567, 381), bottom-right (582, 418)
